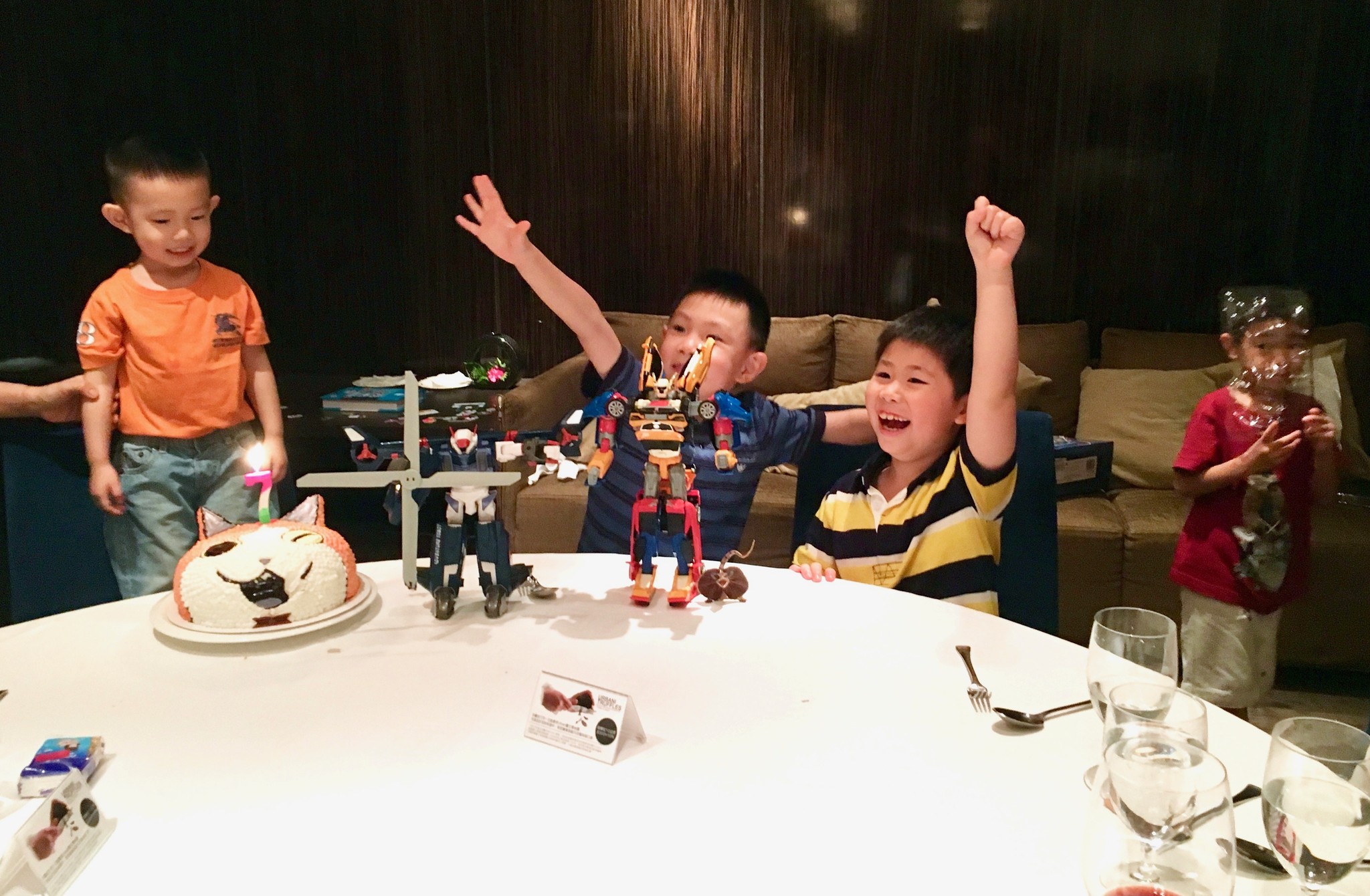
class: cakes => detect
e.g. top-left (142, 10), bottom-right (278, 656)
top-left (165, 491), bottom-right (359, 629)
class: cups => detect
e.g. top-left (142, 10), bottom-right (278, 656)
top-left (1085, 734), bottom-right (1237, 896)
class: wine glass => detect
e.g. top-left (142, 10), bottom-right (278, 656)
top-left (1083, 606), bottom-right (1178, 792)
top-left (1261, 716), bottom-right (1370, 896)
top-left (1103, 683), bottom-right (1208, 882)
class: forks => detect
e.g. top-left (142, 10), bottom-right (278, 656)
top-left (956, 645), bottom-right (992, 713)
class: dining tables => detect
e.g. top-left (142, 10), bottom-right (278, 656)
top-left (0, 542), bottom-right (1370, 896)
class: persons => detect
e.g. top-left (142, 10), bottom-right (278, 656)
top-left (1173, 279), bottom-right (1346, 724)
top-left (789, 181), bottom-right (1031, 616)
top-left (78, 127), bottom-right (293, 601)
top-left (456, 169), bottom-right (876, 564)
top-left (0, 373), bottom-right (120, 423)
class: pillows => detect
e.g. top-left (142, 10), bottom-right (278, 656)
top-left (1073, 338), bottom-right (1368, 490)
top-left (763, 352), bottom-right (1049, 447)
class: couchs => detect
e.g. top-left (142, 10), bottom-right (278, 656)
top-left (488, 308), bottom-right (1370, 675)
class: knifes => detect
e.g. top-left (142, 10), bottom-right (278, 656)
top-left (1155, 784), bottom-right (1264, 854)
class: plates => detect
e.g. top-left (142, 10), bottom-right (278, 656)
top-left (418, 377), bottom-right (473, 389)
top-left (352, 376), bottom-right (405, 388)
top-left (150, 571), bottom-right (378, 644)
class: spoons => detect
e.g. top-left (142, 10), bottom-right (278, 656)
top-left (1235, 838), bottom-right (1370, 875)
top-left (993, 700), bottom-right (1092, 727)
